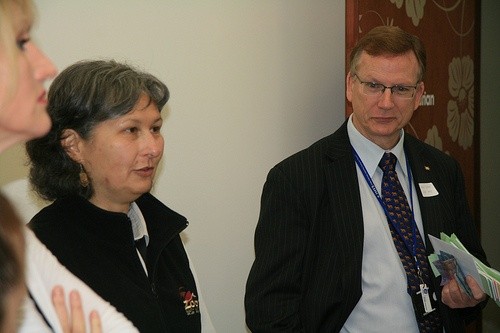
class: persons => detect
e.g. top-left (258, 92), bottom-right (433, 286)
top-left (0, 2), bottom-right (142, 333)
top-left (25, 60), bottom-right (202, 333)
top-left (244, 25), bottom-right (491, 333)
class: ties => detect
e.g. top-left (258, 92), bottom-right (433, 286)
top-left (378, 152), bottom-right (443, 333)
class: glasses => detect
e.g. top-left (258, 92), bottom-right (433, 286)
top-left (355, 73), bottom-right (419, 98)
